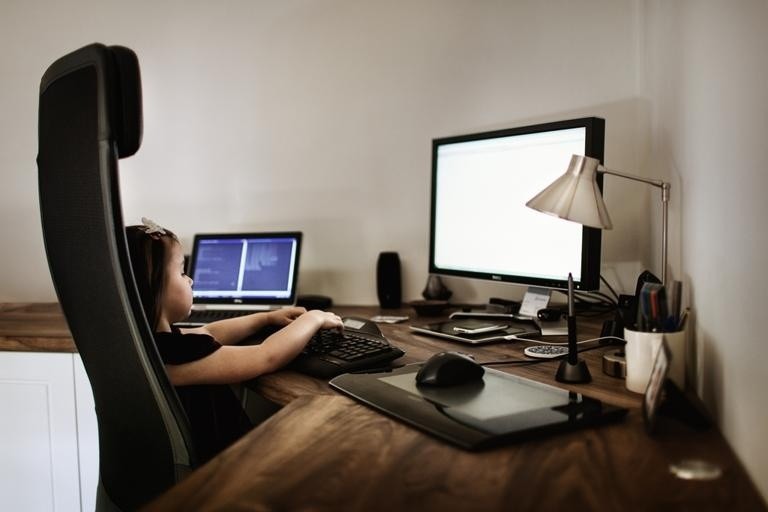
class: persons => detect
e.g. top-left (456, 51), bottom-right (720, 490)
top-left (127, 219), bottom-right (345, 470)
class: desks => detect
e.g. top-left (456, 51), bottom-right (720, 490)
top-left (1, 291), bottom-right (768, 510)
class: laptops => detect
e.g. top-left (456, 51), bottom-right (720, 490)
top-left (171, 231), bottom-right (304, 328)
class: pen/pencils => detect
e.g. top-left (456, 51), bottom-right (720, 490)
top-left (568, 273), bottom-right (577, 365)
top-left (638, 280), bottom-right (691, 333)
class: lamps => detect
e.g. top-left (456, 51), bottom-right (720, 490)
top-left (523, 152), bottom-right (675, 290)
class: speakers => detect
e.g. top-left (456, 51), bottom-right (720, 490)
top-left (633, 270), bottom-right (663, 330)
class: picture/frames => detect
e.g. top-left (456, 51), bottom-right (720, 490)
top-left (639, 331), bottom-right (675, 433)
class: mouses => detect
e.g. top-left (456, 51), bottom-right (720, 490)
top-left (415, 352), bottom-right (484, 387)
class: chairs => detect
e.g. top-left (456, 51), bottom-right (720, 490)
top-left (36, 42), bottom-right (213, 510)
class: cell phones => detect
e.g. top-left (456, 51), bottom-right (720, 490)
top-left (454, 321), bottom-right (508, 334)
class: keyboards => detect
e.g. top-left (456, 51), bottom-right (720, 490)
top-left (236, 316), bottom-right (405, 381)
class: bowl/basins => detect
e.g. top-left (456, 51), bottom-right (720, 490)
top-left (408, 300), bottom-right (451, 316)
top-left (298, 293), bottom-right (332, 311)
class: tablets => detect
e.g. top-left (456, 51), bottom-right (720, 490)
top-left (408, 320), bottom-right (540, 345)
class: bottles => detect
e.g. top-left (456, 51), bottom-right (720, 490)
top-left (599, 320), bottom-right (634, 346)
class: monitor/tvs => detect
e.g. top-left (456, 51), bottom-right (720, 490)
top-left (429, 117), bottom-right (605, 335)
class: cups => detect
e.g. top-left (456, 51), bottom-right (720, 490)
top-left (624, 323), bottom-right (688, 394)
